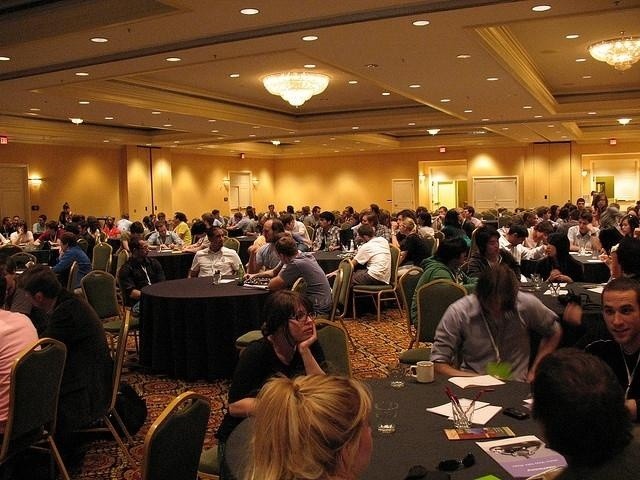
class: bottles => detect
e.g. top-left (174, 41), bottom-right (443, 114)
top-left (237, 264), bottom-right (246, 286)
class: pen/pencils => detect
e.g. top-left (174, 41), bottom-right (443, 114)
top-left (548, 279), bottom-right (560, 293)
top-left (442, 384), bottom-right (482, 426)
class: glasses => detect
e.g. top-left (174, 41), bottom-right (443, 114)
top-left (288, 311), bottom-right (317, 322)
top-left (436, 453), bottom-right (475, 472)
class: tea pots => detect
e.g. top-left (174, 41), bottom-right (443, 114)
top-left (341, 239), bottom-right (356, 252)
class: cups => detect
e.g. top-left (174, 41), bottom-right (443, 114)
top-left (409, 361), bottom-right (436, 385)
top-left (388, 362), bottom-right (407, 389)
top-left (577, 238), bottom-right (601, 260)
top-left (532, 276), bottom-right (561, 298)
top-left (375, 400), bottom-right (399, 435)
top-left (452, 400), bottom-right (475, 430)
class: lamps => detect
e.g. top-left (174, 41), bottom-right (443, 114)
top-left (261, 71), bottom-right (332, 109)
top-left (588, 35), bottom-right (640, 73)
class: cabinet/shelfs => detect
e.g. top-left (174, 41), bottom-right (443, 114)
top-left (522, 142), bottom-right (580, 211)
top-left (119, 145), bottom-right (173, 225)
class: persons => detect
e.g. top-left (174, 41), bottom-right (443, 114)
top-left (1, 189), bottom-right (639, 480)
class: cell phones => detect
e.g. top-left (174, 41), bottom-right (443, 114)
top-left (504, 408), bottom-right (529, 419)
